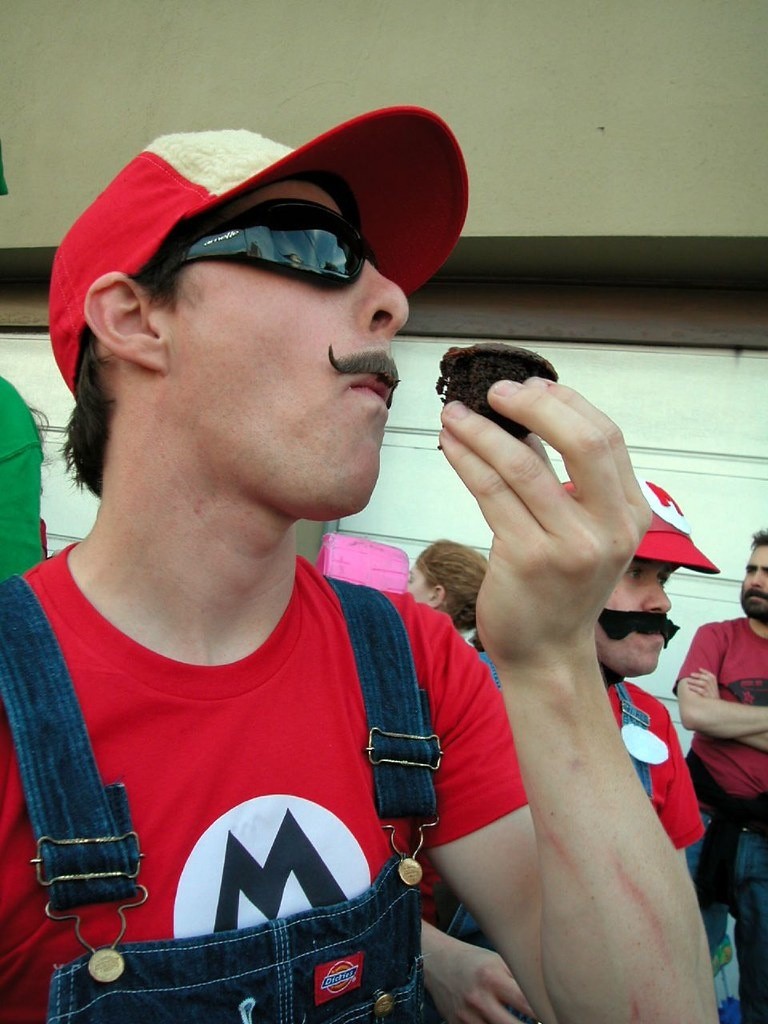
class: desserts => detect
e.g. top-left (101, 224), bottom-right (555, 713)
top-left (433, 344), bottom-right (559, 443)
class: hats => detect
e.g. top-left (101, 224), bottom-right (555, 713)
top-left (49, 105), bottom-right (469, 402)
top-left (560, 477), bottom-right (721, 575)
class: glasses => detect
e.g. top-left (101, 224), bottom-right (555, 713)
top-left (169, 198), bottom-right (381, 289)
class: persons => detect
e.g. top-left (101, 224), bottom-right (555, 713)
top-left (314, 476), bottom-right (767, 1024)
top-left (0, 106), bottom-right (718, 1024)
top-left (1, 375), bottom-right (47, 582)
